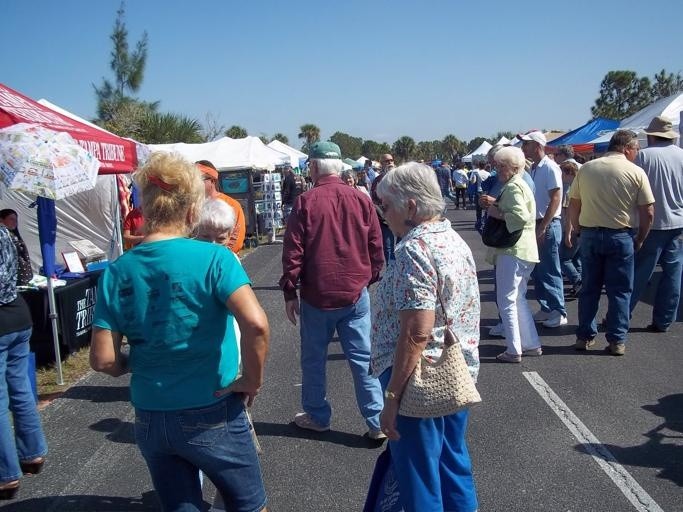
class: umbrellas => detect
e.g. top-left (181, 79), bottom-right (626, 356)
top-left (0, 123), bottom-right (97, 198)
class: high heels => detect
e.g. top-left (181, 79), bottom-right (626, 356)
top-left (0, 481), bottom-right (21, 499)
top-left (19, 459), bottom-right (45, 473)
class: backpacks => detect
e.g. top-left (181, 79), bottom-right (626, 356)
top-left (294, 175), bottom-right (307, 193)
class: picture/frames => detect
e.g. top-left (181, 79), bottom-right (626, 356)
top-left (62, 251), bottom-right (85, 274)
top-left (67, 238), bottom-right (103, 257)
top-left (220, 170), bottom-right (249, 194)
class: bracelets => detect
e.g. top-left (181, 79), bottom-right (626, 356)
top-left (385, 389), bottom-right (401, 401)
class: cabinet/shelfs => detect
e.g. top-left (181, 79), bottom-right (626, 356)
top-left (260, 170), bottom-right (284, 244)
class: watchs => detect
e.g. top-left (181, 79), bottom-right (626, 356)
top-left (499, 211), bottom-right (503, 220)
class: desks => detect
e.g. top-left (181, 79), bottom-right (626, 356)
top-left (20, 274), bottom-right (102, 355)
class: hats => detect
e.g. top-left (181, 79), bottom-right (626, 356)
top-left (642, 115), bottom-right (678, 139)
top-left (516, 131), bottom-right (547, 147)
top-left (304, 141), bottom-right (342, 165)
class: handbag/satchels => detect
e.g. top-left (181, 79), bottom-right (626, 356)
top-left (481, 182), bottom-right (524, 248)
top-left (398, 238), bottom-right (483, 419)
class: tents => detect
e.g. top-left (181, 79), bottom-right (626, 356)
top-left (0, 84), bottom-right (137, 386)
top-left (356, 155), bottom-right (381, 169)
top-left (545, 116), bottom-right (619, 154)
top-left (267, 140), bottom-right (310, 168)
top-left (588, 90), bottom-right (683, 149)
top-left (139, 136), bottom-right (290, 243)
top-left (344, 157), bottom-right (363, 169)
top-left (342, 154), bottom-right (352, 172)
top-left (510, 134), bottom-right (522, 147)
top-left (462, 141), bottom-right (493, 162)
top-left (496, 136), bottom-right (508, 144)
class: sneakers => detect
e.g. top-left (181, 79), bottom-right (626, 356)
top-left (574, 337), bottom-right (596, 350)
top-left (605, 341), bottom-right (625, 355)
top-left (544, 310), bottom-right (568, 328)
top-left (532, 310), bottom-right (550, 323)
top-left (490, 323), bottom-right (507, 337)
top-left (521, 347), bottom-right (543, 357)
top-left (496, 351), bottom-right (523, 363)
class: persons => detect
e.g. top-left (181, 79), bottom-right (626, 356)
top-left (370, 154), bottom-right (402, 267)
top-left (596, 116), bottom-right (683, 333)
top-left (341, 169), bottom-right (371, 199)
top-left (567, 129), bottom-right (656, 355)
top-left (557, 144), bottom-right (583, 172)
top-left (473, 161), bottom-right (492, 228)
top-left (368, 160), bottom-right (481, 512)
top-left (0, 225), bottom-right (47, 501)
top-left (0, 209), bottom-right (34, 284)
top-left (365, 160), bottom-right (375, 184)
top-left (559, 162), bottom-right (582, 297)
top-left (374, 168), bottom-right (381, 177)
top-left (487, 146), bottom-right (543, 364)
top-left (452, 163), bottom-right (469, 210)
top-left (358, 170), bottom-right (370, 190)
top-left (196, 161), bottom-right (246, 256)
top-left (574, 154), bottom-right (585, 163)
top-left (479, 145), bottom-right (536, 339)
top-left (485, 164), bottom-right (491, 172)
top-left (89, 153), bottom-right (270, 512)
top-left (278, 142), bottom-right (391, 440)
top-left (435, 162), bottom-right (457, 215)
top-left (547, 153), bottom-right (555, 161)
top-left (281, 165), bottom-right (295, 227)
top-left (467, 166), bottom-right (478, 206)
top-left (122, 180), bottom-right (147, 249)
top-left (192, 198), bottom-right (261, 512)
top-left (516, 130), bottom-right (568, 327)
